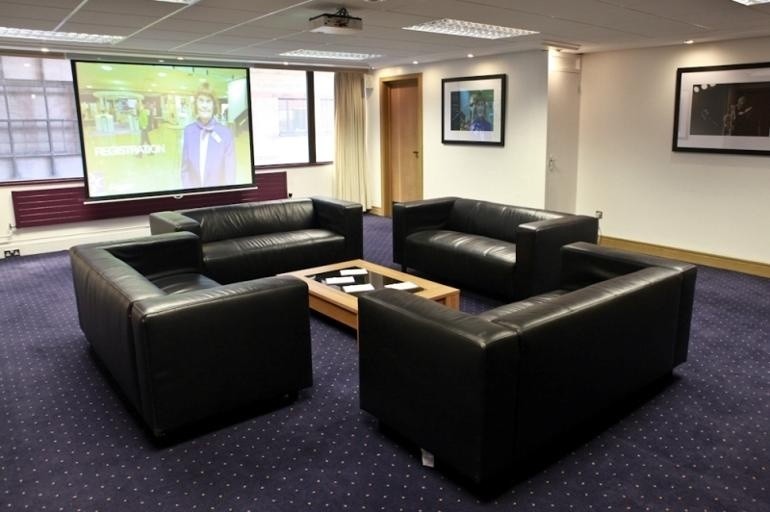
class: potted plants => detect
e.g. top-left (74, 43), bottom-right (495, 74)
top-left (673, 61), bottom-right (770, 155)
top-left (441, 74), bottom-right (505, 145)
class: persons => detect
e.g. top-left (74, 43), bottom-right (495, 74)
top-left (181, 88), bottom-right (237, 188)
top-left (134, 101), bottom-right (158, 154)
top-left (725, 96), bottom-right (759, 136)
top-left (468, 99), bottom-right (491, 131)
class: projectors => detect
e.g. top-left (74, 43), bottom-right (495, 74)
top-left (307, 13), bottom-right (363, 35)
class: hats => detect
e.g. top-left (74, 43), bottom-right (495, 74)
top-left (194, 86), bottom-right (218, 114)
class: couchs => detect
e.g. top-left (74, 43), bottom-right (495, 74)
top-left (393, 195), bottom-right (598, 297)
top-left (358, 241), bottom-right (695, 503)
top-left (69, 231), bottom-right (311, 452)
top-left (150, 196), bottom-right (363, 288)
